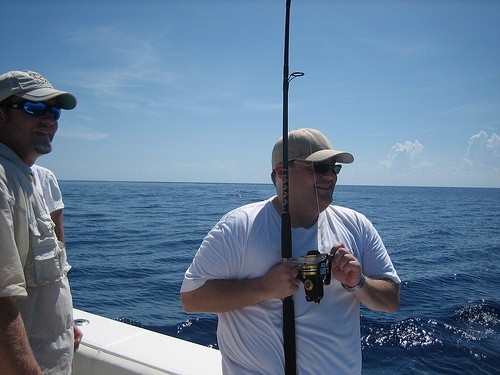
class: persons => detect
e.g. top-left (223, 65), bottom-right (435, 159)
top-left (30, 164), bottom-right (64, 243)
top-left (180, 128), bottom-right (401, 375)
top-left (0, 70), bottom-right (83, 375)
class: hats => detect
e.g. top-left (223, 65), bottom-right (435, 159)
top-left (0, 70), bottom-right (77, 110)
top-left (272, 128), bottom-right (354, 163)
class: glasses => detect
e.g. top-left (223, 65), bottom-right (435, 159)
top-left (9, 102), bottom-right (62, 120)
top-left (290, 161), bottom-right (342, 176)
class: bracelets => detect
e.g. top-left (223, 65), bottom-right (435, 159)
top-left (341, 273), bottom-right (366, 292)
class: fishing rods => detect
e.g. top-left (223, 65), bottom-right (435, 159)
top-left (281, 0), bottom-right (335, 375)
